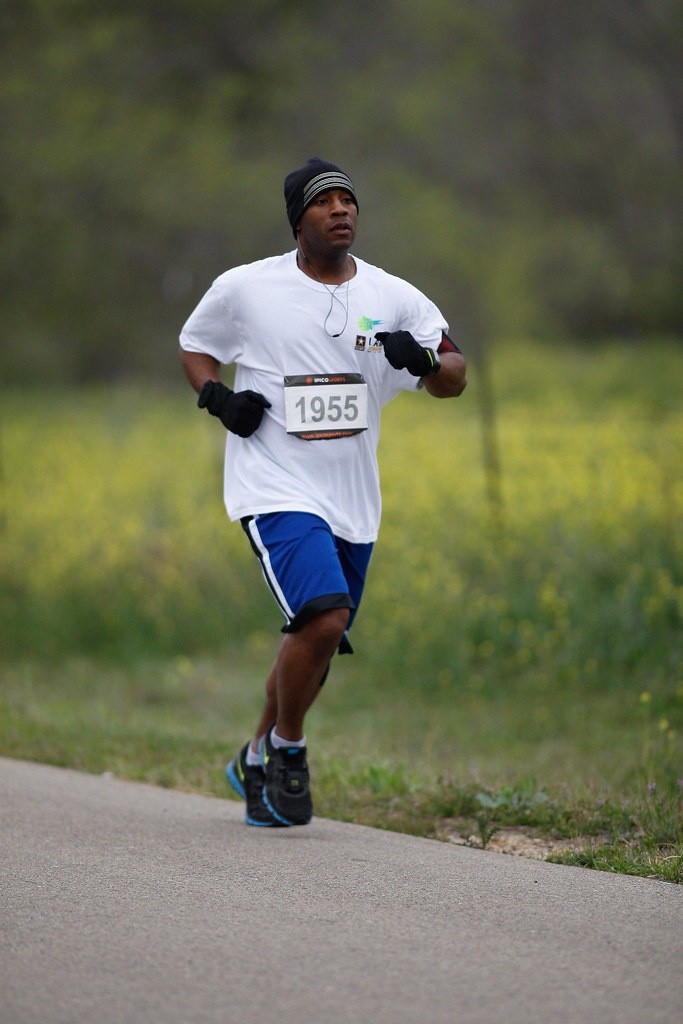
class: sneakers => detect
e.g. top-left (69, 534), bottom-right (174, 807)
top-left (257, 724), bottom-right (313, 825)
top-left (226, 740), bottom-right (272, 826)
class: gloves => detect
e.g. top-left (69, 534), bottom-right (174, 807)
top-left (197, 380), bottom-right (272, 438)
top-left (375, 330), bottom-right (441, 382)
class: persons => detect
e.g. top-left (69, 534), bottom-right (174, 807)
top-left (179, 155), bottom-right (468, 825)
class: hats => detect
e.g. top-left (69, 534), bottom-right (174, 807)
top-left (283, 157), bottom-right (359, 241)
